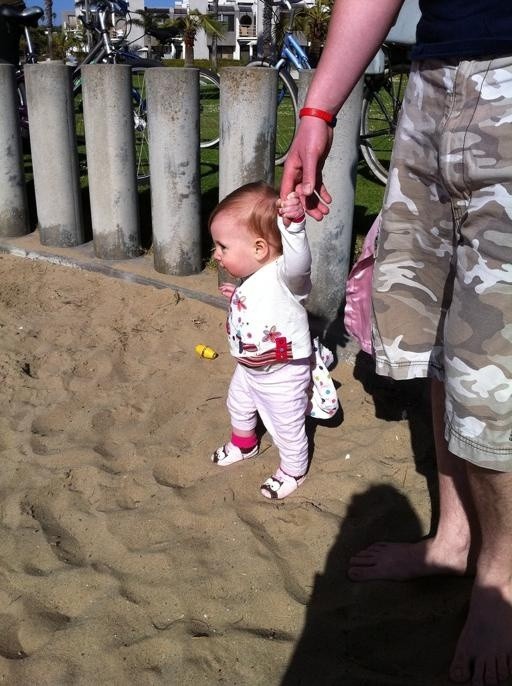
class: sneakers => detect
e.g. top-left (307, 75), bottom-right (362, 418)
top-left (211, 441), bottom-right (259, 466)
top-left (260, 467), bottom-right (306, 500)
